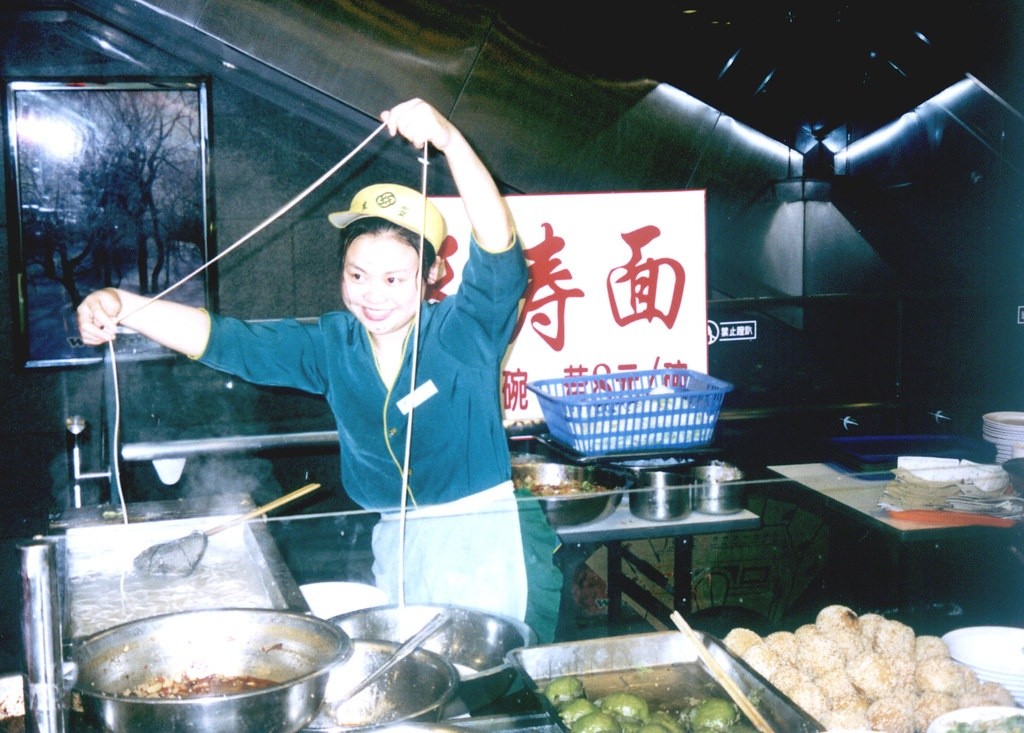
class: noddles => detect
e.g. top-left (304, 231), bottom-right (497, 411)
top-left (103, 123), bottom-right (428, 608)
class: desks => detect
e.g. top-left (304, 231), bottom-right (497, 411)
top-left (554, 497), bottom-right (762, 637)
top-left (768, 462), bottom-right (1024, 631)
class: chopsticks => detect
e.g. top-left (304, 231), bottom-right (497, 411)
top-left (671, 612), bottom-right (776, 733)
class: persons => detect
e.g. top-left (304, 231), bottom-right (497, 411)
top-left (76, 96), bottom-right (565, 697)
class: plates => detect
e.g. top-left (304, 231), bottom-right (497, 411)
top-left (941, 625), bottom-right (1023, 702)
top-left (982, 411), bottom-right (1024, 464)
top-left (928, 705), bottom-right (1024, 733)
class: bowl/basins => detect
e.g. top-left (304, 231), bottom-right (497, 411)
top-left (630, 471), bottom-right (690, 520)
top-left (508, 462), bottom-right (633, 528)
top-left (63, 608), bottom-right (352, 733)
top-left (322, 601), bottom-right (540, 717)
top-left (302, 637), bottom-right (461, 733)
top-left (689, 464), bottom-right (745, 515)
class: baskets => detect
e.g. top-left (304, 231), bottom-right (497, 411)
top-left (526, 369), bottom-right (734, 458)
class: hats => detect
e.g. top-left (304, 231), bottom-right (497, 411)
top-left (327, 183), bottom-right (446, 258)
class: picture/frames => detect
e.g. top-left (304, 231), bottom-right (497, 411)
top-left (1, 75), bottom-right (218, 371)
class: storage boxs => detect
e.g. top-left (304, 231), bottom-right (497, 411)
top-left (526, 369), bottom-right (733, 457)
